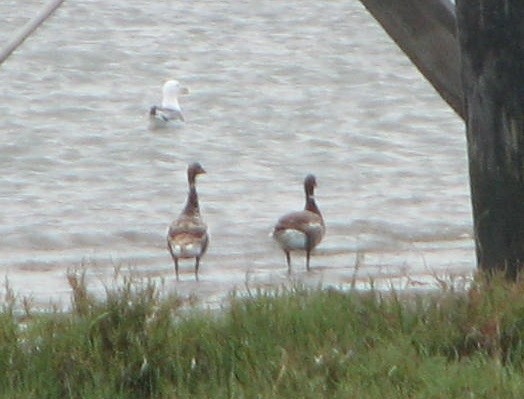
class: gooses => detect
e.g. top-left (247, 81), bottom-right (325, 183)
top-left (148, 80), bottom-right (190, 129)
top-left (167, 162), bottom-right (209, 283)
top-left (271, 175), bottom-right (325, 277)
